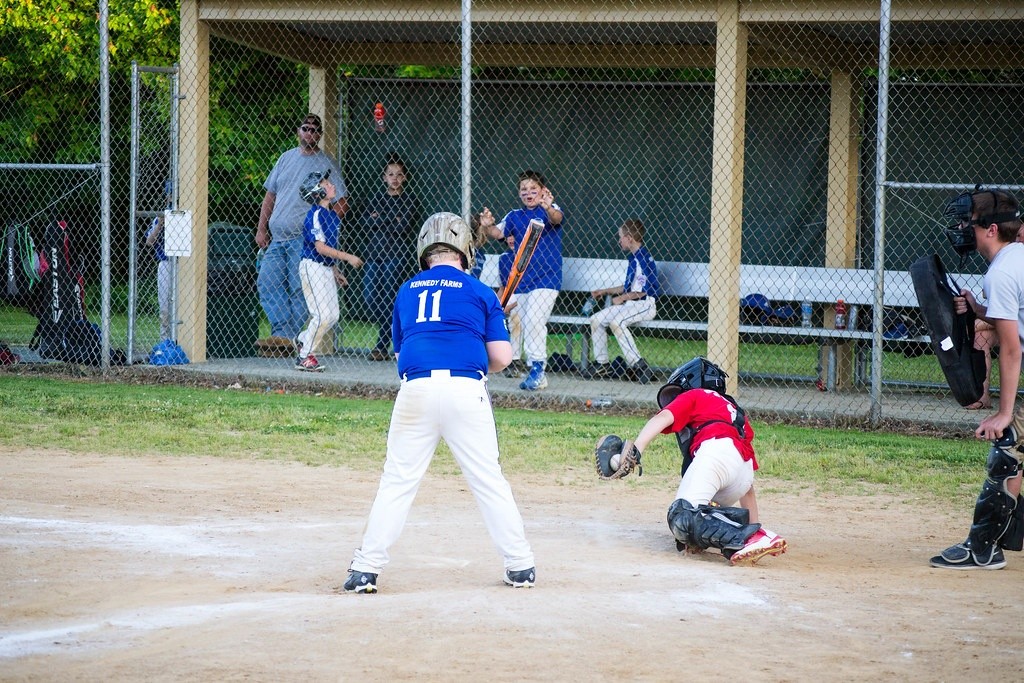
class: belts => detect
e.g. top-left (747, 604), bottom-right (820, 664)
top-left (693, 435), bottom-right (728, 456)
top-left (407, 370), bottom-right (481, 380)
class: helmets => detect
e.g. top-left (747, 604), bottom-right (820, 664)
top-left (417, 212), bottom-right (475, 269)
top-left (741, 294), bottom-right (771, 322)
top-left (300, 169), bottom-right (331, 205)
top-left (658, 358), bottom-right (726, 408)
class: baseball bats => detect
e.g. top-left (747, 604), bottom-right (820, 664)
top-left (501, 219), bottom-right (545, 310)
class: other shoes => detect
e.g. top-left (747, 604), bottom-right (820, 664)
top-left (369, 351), bottom-right (389, 361)
top-left (636, 366), bottom-right (651, 384)
top-left (581, 364), bottom-right (610, 378)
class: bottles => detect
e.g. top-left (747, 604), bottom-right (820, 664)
top-left (835, 297), bottom-right (846, 330)
top-left (802, 296), bottom-right (813, 328)
top-left (586, 397), bottom-right (613, 409)
top-left (848, 306), bottom-right (857, 331)
top-left (581, 295), bottom-right (596, 315)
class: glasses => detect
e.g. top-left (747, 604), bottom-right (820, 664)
top-left (302, 126), bottom-right (316, 133)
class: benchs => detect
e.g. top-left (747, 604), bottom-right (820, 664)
top-left (480, 254), bottom-right (1024, 398)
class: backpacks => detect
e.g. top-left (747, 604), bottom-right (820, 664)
top-left (0, 220), bottom-right (117, 365)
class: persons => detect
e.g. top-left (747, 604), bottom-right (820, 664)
top-left (344, 211), bottom-right (537, 596)
top-left (928, 189), bottom-right (1024, 571)
top-left (144, 177), bottom-right (174, 343)
top-left (360, 150), bottom-right (419, 363)
top-left (255, 113), bottom-right (350, 349)
top-left (292, 169), bottom-right (364, 373)
top-left (596, 358), bottom-right (787, 567)
top-left (470, 168), bottom-right (564, 389)
top-left (577, 218), bottom-right (657, 381)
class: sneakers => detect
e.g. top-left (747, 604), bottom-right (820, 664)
top-left (344, 568), bottom-right (379, 594)
top-left (519, 370), bottom-right (548, 389)
top-left (729, 528), bottom-right (787, 567)
top-left (504, 567), bottom-right (535, 588)
top-left (296, 355), bottom-right (325, 372)
top-left (293, 336), bottom-right (304, 354)
top-left (931, 547), bottom-right (1006, 570)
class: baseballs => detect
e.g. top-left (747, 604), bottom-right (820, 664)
top-left (609, 454), bottom-right (621, 471)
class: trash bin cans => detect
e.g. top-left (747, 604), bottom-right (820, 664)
top-left (207, 221), bottom-right (261, 357)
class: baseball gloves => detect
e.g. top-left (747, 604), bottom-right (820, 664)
top-left (593, 431), bottom-right (643, 480)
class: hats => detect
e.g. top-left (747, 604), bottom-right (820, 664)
top-left (300, 113), bottom-right (322, 130)
